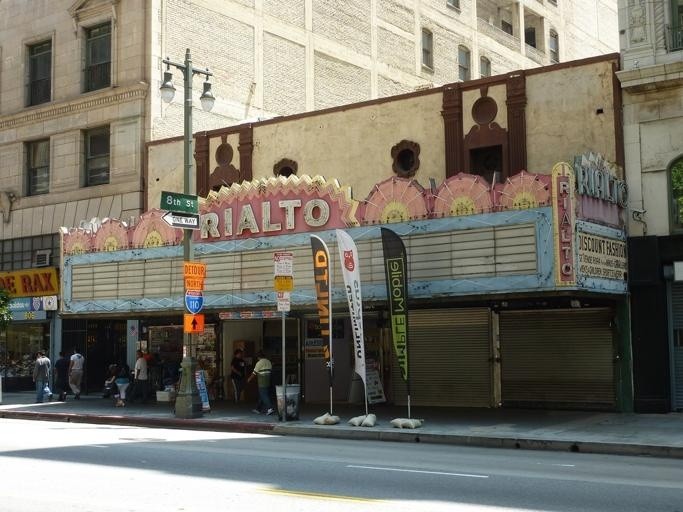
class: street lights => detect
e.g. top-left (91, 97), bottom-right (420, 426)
top-left (158, 46), bottom-right (217, 422)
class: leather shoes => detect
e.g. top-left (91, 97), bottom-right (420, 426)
top-left (76, 392), bottom-right (81, 400)
top-left (47, 393), bottom-right (54, 402)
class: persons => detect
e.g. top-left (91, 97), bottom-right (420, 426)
top-left (130, 349), bottom-right (148, 403)
top-left (103, 357), bottom-right (131, 407)
top-left (230, 348), bottom-right (245, 409)
top-left (54, 351), bottom-right (68, 402)
top-left (66, 347), bottom-right (84, 401)
top-left (246, 352), bottom-right (276, 416)
top-left (31, 349), bottom-right (52, 403)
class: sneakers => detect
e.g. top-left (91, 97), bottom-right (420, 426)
top-left (251, 408), bottom-right (260, 414)
top-left (265, 408), bottom-right (275, 416)
top-left (58, 392), bottom-right (67, 402)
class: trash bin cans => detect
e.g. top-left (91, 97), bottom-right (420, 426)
top-left (277, 384), bottom-right (301, 421)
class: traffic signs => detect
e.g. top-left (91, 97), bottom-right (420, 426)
top-left (161, 211), bottom-right (202, 231)
top-left (160, 190), bottom-right (200, 217)
